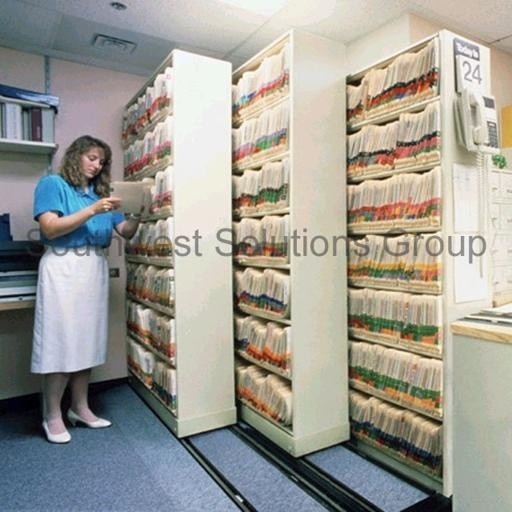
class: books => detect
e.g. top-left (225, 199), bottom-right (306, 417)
top-left (0, 102), bottom-right (42, 142)
top-left (232, 42), bottom-right (293, 427)
top-left (120, 67), bottom-right (177, 418)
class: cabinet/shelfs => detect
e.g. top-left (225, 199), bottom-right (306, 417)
top-left (0, 93), bottom-right (62, 156)
top-left (119, 25), bottom-right (494, 500)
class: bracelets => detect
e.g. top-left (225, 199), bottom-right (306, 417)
top-left (130, 213), bottom-right (140, 221)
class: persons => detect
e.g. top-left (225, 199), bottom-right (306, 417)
top-left (33, 135), bottom-right (145, 444)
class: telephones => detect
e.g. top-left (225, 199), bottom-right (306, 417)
top-left (458, 86), bottom-right (501, 157)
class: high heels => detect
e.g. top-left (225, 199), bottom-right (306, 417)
top-left (42, 420), bottom-right (71, 443)
top-left (67, 408), bottom-right (112, 429)
